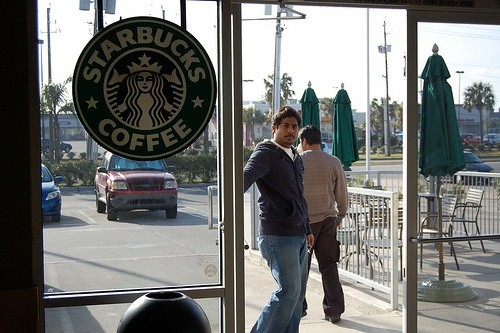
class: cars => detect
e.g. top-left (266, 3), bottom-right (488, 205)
top-left (40, 138), bottom-right (72, 153)
top-left (459, 132), bottom-right (500, 150)
top-left (424, 151), bottom-right (494, 185)
top-left (41, 164), bottom-right (63, 222)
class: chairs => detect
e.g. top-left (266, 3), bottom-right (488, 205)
top-left (339, 185), bottom-right (485, 281)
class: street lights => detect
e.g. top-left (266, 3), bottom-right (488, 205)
top-left (38, 39), bottom-right (45, 156)
top-left (456, 71), bottom-right (464, 127)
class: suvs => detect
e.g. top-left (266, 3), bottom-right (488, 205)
top-left (94, 151), bottom-right (178, 221)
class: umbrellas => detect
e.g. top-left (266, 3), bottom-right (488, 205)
top-left (332, 83), bottom-right (359, 171)
top-left (419, 43), bottom-right (466, 248)
top-left (300, 81), bottom-right (326, 151)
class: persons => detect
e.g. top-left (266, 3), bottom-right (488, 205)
top-left (244, 106), bottom-right (315, 333)
top-left (297, 124), bottom-right (348, 322)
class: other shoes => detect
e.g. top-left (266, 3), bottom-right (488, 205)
top-left (325, 313), bottom-right (341, 322)
top-left (302, 309), bottom-right (307, 317)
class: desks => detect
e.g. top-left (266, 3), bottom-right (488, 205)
top-left (341, 206), bottom-right (375, 275)
top-left (370, 199), bottom-right (402, 237)
top-left (419, 192), bottom-right (453, 229)
